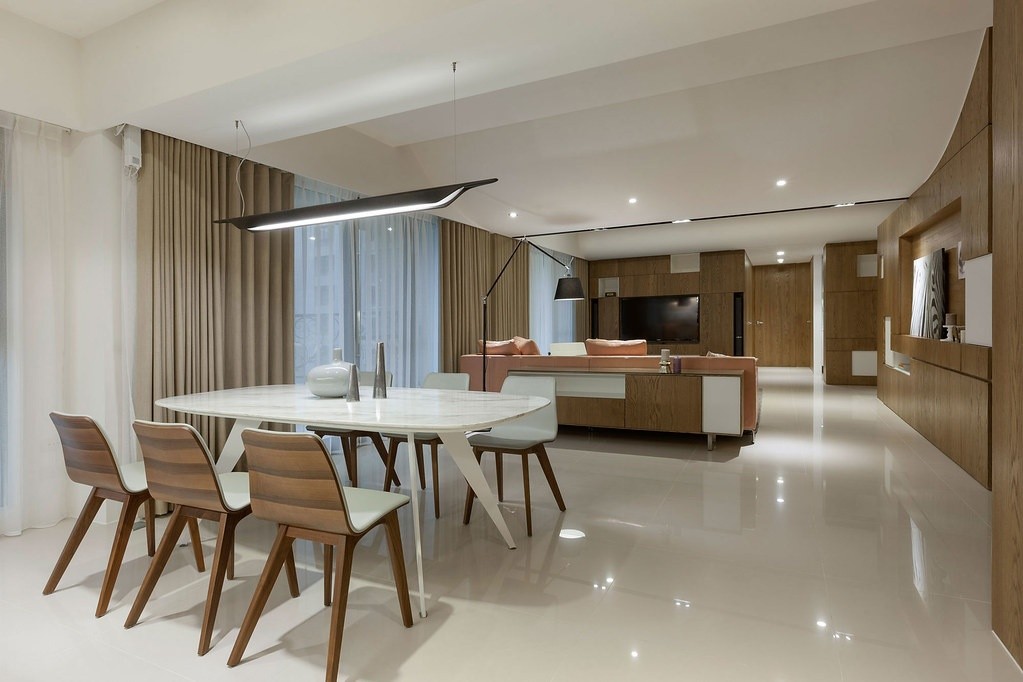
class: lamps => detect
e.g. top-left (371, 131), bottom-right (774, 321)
top-left (480, 238), bottom-right (586, 392)
top-left (212, 62), bottom-right (498, 233)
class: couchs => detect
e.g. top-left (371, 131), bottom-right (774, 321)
top-left (459, 352), bottom-right (765, 452)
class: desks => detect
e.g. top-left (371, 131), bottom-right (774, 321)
top-left (153, 384), bottom-right (551, 618)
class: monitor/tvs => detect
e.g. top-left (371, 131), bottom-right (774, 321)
top-left (619, 294), bottom-right (700, 343)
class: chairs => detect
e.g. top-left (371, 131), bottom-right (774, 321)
top-left (305, 370), bottom-right (401, 489)
top-left (41, 411), bottom-right (206, 617)
top-left (463, 375), bottom-right (567, 538)
top-left (225, 427), bottom-right (411, 682)
top-left (123, 418), bottom-right (301, 656)
top-left (383, 371), bottom-right (480, 520)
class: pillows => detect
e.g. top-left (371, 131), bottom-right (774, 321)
top-left (511, 335), bottom-right (540, 355)
top-left (477, 338), bottom-right (521, 356)
top-left (585, 338), bottom-right (647, 356)
